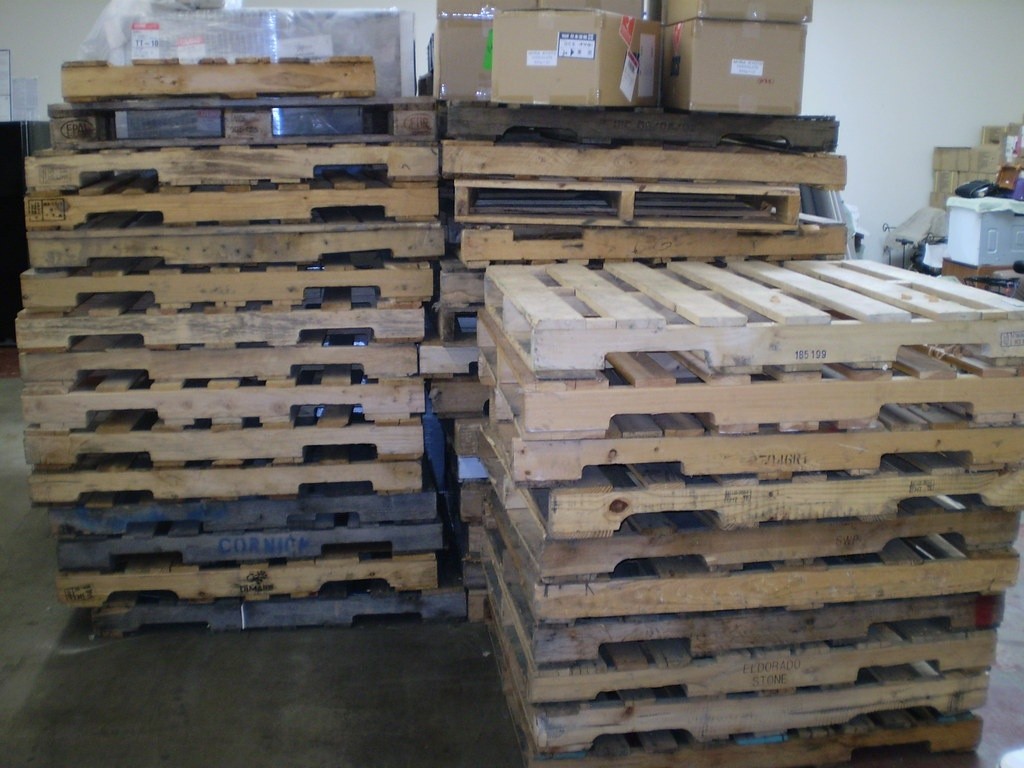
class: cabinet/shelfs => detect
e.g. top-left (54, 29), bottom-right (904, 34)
top-left (941, 256), bottom-right (1014, 299)
top-left (0, 119), bottom-right (51, 349)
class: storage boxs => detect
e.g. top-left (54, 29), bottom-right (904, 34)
top-left (994, 168), bottom-right (1024, 202)
top-left (982, 125), bottom-right (1023, 146)
top-left (933, 169), bottom-right (997, 194)
top-left (274, 6), bottom-right (401, 138)
top-left (933, 146), bottom-right (1001, 175)
top-left (666, 0), bottom-right (814, 23)
top-left (122, 8), bottom-right (274, 142)
top-left (433, 0), bottom-right (643, 102)
top-left (946, 196), bottom-right (1024, 266)
top-left (491, 8), bottom-right (662, 106)
top-left (930, 192), bottom-right (948, 209)
top-left (660, 18), bottom-right (807, 116)
